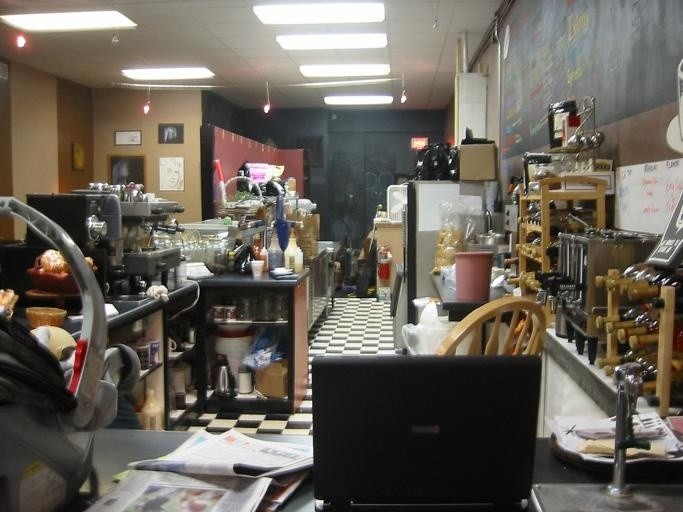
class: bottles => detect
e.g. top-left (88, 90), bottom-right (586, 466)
top-left (285, 176), bottom-right (297, 196)
top-left (284, 229), bottom-right (299, 270)
top-left (502, 254), bottom-right (520, 287)
top-left (153, 233), bottom-right (229, 265)
top-left (268, 228), bottom-right (284, 271)
top-left (257, 245), bottom-right (268, 272)
top-left (587, 260), bottom-right (683, 410)
top-left (237, 364), bottom-right (253, 394)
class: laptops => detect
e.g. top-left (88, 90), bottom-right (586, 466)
top-left (312, 355), bottom-right (542, 512)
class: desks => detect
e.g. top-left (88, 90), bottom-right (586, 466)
top-left (63, 430), bottom-right (683, 512)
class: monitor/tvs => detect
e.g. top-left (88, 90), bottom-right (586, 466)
top-left (26, 195), bottom-right (86, 248)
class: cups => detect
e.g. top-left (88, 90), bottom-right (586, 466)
top-left (211, 294), bottom-right (290, 322)
top-left (173, 391), bottom-right (186, 410)
top-left (251, 260), bottom-right (265, 279)
top-left (168, 336), bottom-right (177, 357)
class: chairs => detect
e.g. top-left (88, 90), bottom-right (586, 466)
top-left (437, 295), bottom-right (546, 364)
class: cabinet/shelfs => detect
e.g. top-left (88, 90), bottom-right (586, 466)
top-left (516, 176), bottom-right (609, 329)
top-left (58, 266), bottom-right (313, 431)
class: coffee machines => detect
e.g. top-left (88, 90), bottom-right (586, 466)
top-left (24, 192), bottom-right (124, 303)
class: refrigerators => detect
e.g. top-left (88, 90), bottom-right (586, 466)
top-left (403, 179), bottom-right (499, 326)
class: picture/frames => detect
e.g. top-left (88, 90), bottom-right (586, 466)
top-left (105, 130), bottom-right (147, 192)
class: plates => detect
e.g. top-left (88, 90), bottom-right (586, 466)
top-left (547, 410), bottom-right (683, 465)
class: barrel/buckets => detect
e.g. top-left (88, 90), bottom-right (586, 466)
top-left (453, 252), bottom-right (493, 302)
